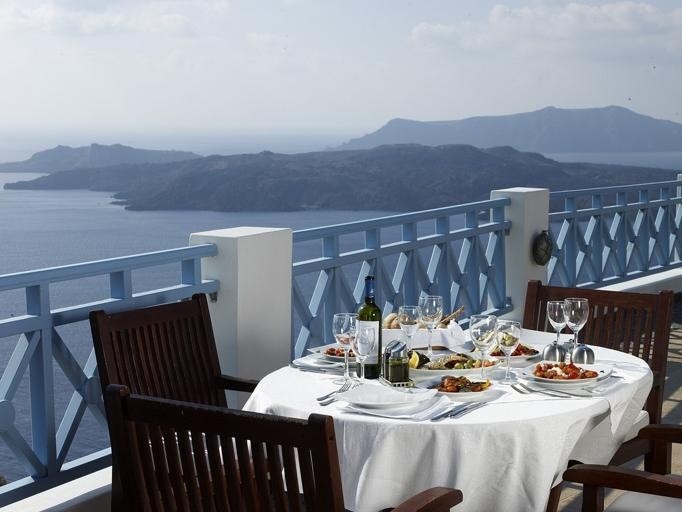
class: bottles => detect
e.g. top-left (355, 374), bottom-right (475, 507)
top-left (355, 273), bottom-right (384, 379)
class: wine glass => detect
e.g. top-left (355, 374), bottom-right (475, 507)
top-left (418, 295), bottom-right (443, 356)
top-left (496, 321), bottom-right (521, 384)
top-left (332, 313), bottom-right (359, 387)
top-left (350, 326), bottom-right (376, 381)
top-left (398, 306), bottom-right (422, 352)
top-left (563, 298), bottom-right (589, 353)
top-left (469, 315), bottom-right (498, 382)
top-left (547, 302), bottom-right (570, 345)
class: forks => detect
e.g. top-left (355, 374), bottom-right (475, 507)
top-left (317, 383), bottom-right (358, 406)
top-left (508, 384), bottom-right (593, 402)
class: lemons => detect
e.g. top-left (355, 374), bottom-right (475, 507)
top-left (409, 350), bottom-right (421, 368)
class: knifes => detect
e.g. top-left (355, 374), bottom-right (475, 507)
top-left (432, 399), bottom-right (489, 424)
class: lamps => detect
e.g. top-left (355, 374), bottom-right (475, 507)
top-left (533, 230), bottom-right (554, 266)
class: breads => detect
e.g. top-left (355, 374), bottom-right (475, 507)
top-left (391, 314), bottom-right (414, 329)
top-left (383, 312), bottom-right (396, 329)
top-left (414, 316), bottom-right (433, 328)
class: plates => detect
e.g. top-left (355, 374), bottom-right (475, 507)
top-left (337, 384), bottom-right (438, 409)
top-left (322, 348), bottom-right (359, 361)
top-left (486, 343), bottom-right (542, 359)
top-left (522, 360), bottom-right (613, 385)
top-left (414, 377), bottom-right (496, 395)
top-left (409, 354), bottom-right (502, 371)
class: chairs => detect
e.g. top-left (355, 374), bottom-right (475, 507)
top-left (88, 293), bottom-right (464, 512)
top-left (521, 278), bottom-right (681, 512)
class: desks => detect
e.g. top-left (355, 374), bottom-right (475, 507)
top-left (217, 325), bottom-right (654, 512)
